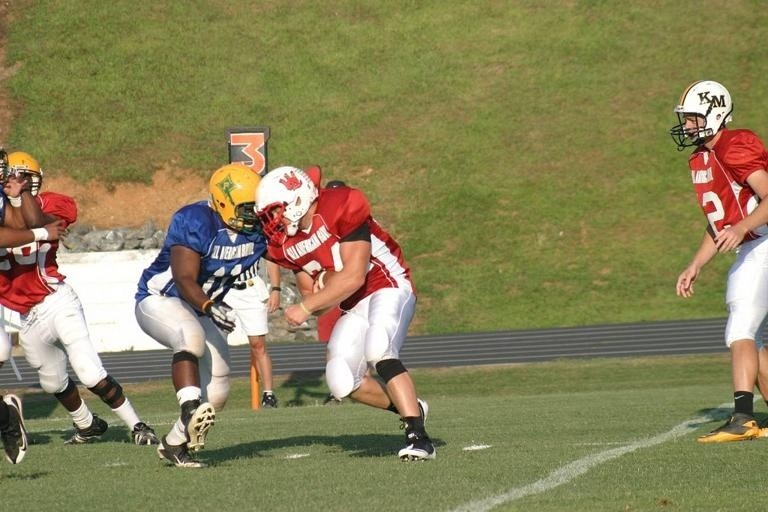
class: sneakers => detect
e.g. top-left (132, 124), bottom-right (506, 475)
top-left (155, 436), bottom-right (208, 470)
top-left (64, 418), bottom-right (108, 443)
top-left (181, 402), bottom-right (216, 453)
top-left (392, 420), bottom-right (439, 461)
top-left (415, 398), bottom-right (430, 426)
top-left (262, 393), bottom-right (278, 410)
top-left (755, 416), bottom-right (768, 439)
top-left (130, 423), bottom-right (158, 444)
top-left (696, 413), bottom-right (760, 444)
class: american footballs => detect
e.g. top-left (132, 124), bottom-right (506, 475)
top-left (313, 270), bottom-right (337, 293)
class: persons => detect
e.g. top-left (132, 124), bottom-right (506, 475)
top-left (0, 153), bottom-right (159, 446)
top-left (669, 78), bottom-right (768, 443)
top-left (223, 260), bottom-right (281, 408)
top-left (0, 146), bottom-right (69, 369)
top-left (254, 166), bottom-right (436, 461)
top-left (323, 180), bottom-right (347, 404)
top-left (134, 163), bottom-right (267, 468)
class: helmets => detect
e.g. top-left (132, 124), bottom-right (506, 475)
top-left (0, 148), bottom-right (9, 186)
top-left (3, 152), bottom-right (43, 209)
top-left (673, 80), bottom-right (734, 145)
top-left (255, 166), bottom-right (320, 246)
top-left (206, 163), bottom-right (262, 236)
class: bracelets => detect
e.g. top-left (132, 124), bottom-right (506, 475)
top-left (202, 300), bottom-right (213, 314)
top-left (300, 302), bottom-right (310, 312)
top-left (300, 288), bottom-right (311, 292)
top-left (7, 196), bottom-right (22, 208)
top-left (30, 227), bottom-right (49, 242)
top-left (272, 286), bottom-right (282, 291)
top-left (20, 188), bottom-right (31, 194)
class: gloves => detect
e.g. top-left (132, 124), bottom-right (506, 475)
top-left (208, 301), bottom-right (237, 332)
